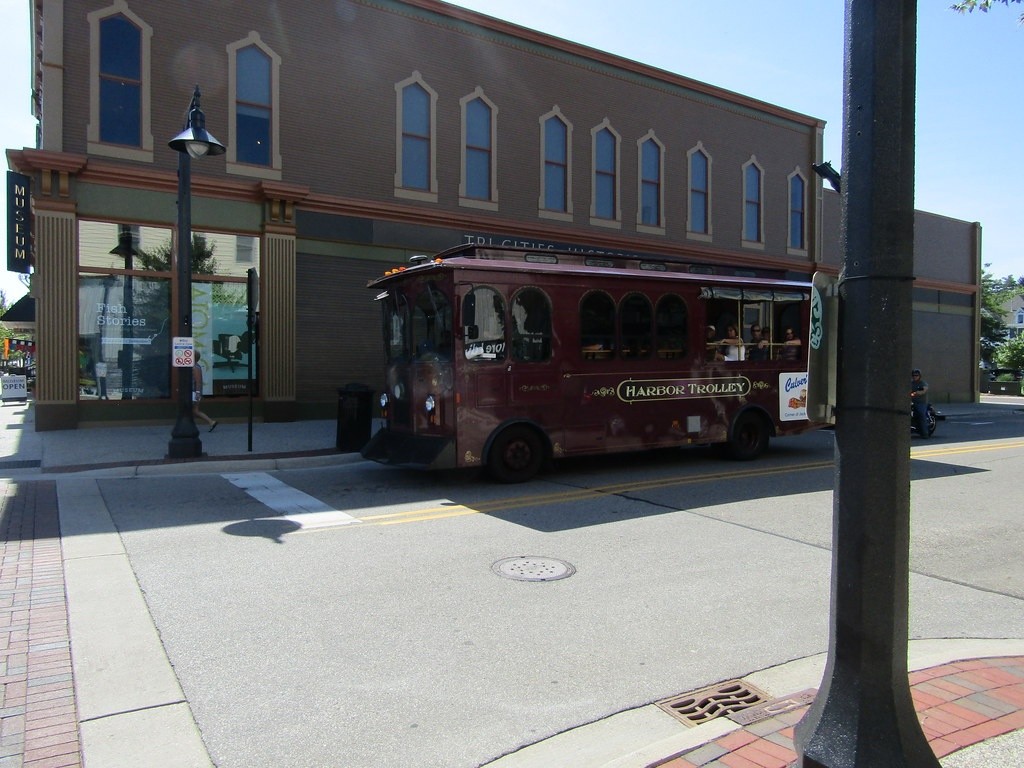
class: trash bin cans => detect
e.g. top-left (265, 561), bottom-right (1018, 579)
top-left (336, 383), bottom-right (376, 452)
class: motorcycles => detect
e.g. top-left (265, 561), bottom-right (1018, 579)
top-left (911, 393), bottom-right (937, 435)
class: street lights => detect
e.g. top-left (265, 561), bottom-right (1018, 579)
top-left (166, 83), bottom-right (227, 461)
top-left (108, 224), bottom-right (140, 400)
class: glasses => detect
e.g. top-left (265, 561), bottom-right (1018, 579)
top-left (912, 375), bottom-right (919, 377)
top-left (785, 332), bottom-right (793, 335)
top-left (751, 330), bottom-right (760, 332)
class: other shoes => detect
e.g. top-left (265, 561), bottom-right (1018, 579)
top-left (921, 432), bottom-right (929, 439)
top-left (208, 420), bottom-right (218, 432)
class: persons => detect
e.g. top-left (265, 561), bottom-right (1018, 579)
top-left (910, 368), bottom-right (930, 439)
top-left (705, 320), bottom-right (802, 361)
top-left (192, 350), bottom-right (219, 432)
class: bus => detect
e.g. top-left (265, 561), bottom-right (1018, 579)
top-left (364, 242), bottom-right (834, 490)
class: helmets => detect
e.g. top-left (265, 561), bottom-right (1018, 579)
top-left (912, 369), bottom-right (920, 375)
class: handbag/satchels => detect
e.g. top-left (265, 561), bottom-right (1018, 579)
top-left (192, 391), bottom-right (201, 402)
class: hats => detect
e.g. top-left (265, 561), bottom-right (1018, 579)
top-left (707, 325), bottom-right (715, 331)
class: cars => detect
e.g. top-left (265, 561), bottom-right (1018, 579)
top-left (991, 369), bottom-right (1023, 381)
